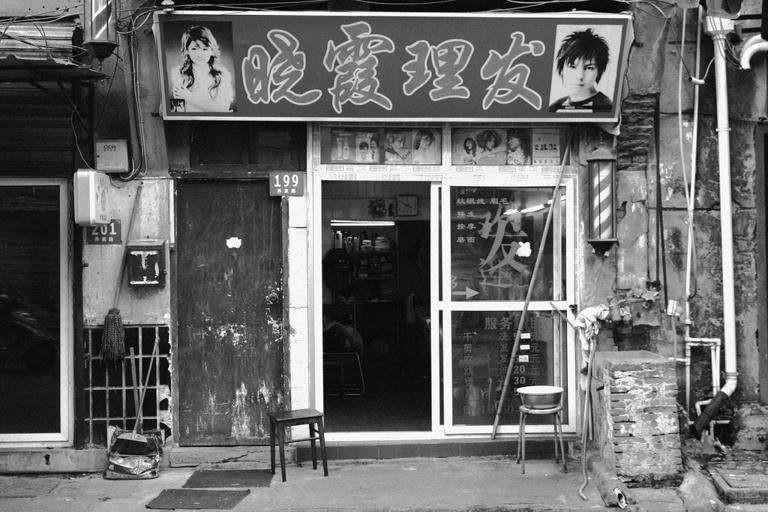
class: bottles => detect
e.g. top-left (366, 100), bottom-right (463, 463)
top-left (334, 230), bottom-right (359, 252)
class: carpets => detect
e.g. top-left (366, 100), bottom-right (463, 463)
top-left (183, 469), bottom-right (273, 487)
top-left (145, 489), bottom-right (250, 510)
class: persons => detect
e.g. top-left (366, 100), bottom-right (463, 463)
top-left (549, 27), bottom-right (613, 112)
top-left (170, 26), bottom-right (233, 115)
top-left (507, 130), bottom-right (531, 164)
top-left (411, 129), bottom-right (434, 163)
top-left (460, 137), bottom-right (477, 164)
top-left (384, 131), bottom-right (411, 164)
top-left (356, 141), bottom-right (372, 161)
top-left (474, 129), bottom-right (508, 165)
top-left (369, 136), bottom-right (380, 162)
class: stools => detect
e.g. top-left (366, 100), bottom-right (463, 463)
top-left (265, 409), bottom-right (328, 482)
top-left (516, 404), bottom-right (567, 473)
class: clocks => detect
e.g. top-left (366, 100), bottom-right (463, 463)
top-left (396, 194), bottom-right (419, 217)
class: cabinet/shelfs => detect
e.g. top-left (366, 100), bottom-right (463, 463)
top-left (333, 225), bottom-right (400, 365)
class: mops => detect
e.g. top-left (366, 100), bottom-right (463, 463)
top-left (101, 183), bottom-right (142, 366)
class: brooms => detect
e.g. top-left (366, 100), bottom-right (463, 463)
top-left (111, 340), bottom-right (158, 454)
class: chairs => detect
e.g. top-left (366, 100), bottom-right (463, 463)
top-left (324, 312), bottom-right (367, 397)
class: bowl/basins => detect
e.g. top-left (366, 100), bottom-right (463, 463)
top-left (514, 383), bottom-right (563, 410)
